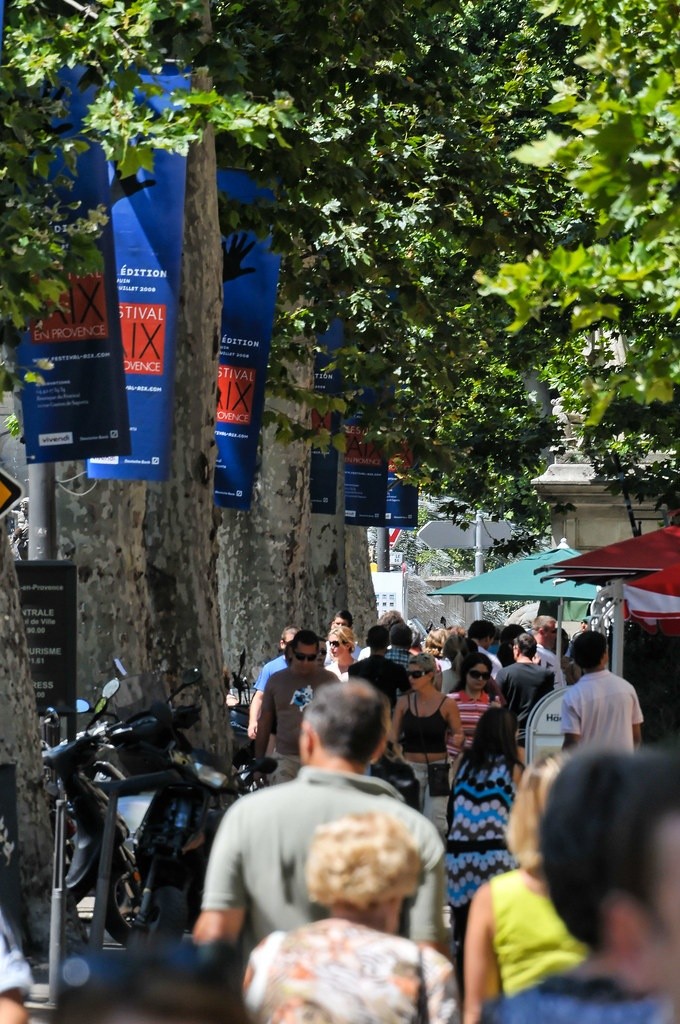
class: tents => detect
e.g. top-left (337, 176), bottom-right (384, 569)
top-left (531, 525), bottom-right (680, 635)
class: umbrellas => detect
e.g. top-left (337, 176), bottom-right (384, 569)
top-left (427, 537), bottom-right (602, 665)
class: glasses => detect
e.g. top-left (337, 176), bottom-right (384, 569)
top-left (294, 650), bottom-right (317, 661)
top-left (468, 670), bottom-right (491, 680)
top-left (407, 669), bottom-right (432, 678)
top-left (328, 641), bottom-right (347, 647)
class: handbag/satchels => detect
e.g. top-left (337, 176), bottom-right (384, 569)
top-left (428, 764), bottom-right (451, 796)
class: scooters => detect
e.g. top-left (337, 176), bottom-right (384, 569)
top-left (34, 647), bottom-right (278, 945)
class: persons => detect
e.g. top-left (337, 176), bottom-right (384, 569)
top-left (0, 908), bottom-right (34, 1024)
top-left (247, 611), bottom-right (644, 860)
top-left (195, 680), bottom-right (449, 957)
top-left (52, 938), bottom-right (248, 1024)
top-left (447, 707), bottom-right (526, 971)
top-left (483, 751), bottom-right (680, 1023)
top-left (462, 755), bottom-right (590, 1024)
top-left (244, 811), bottom-right (460, 1024)
